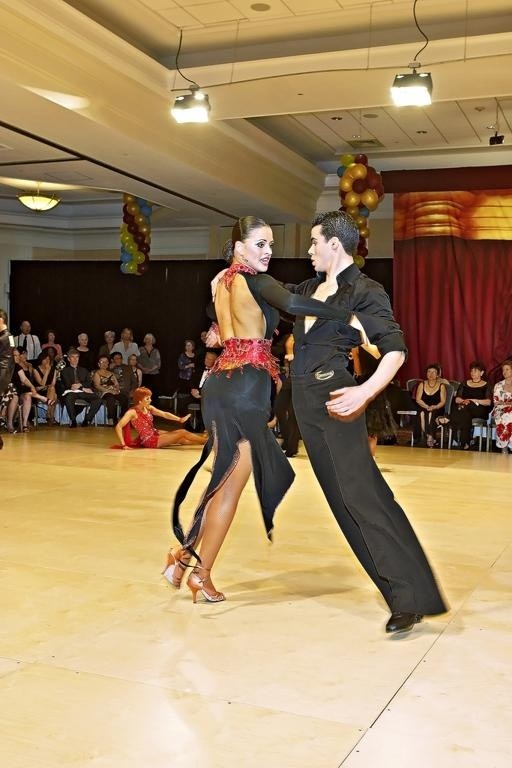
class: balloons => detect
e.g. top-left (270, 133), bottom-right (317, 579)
top-left (334, 153), bottom-right (384, 267)
top-left (118, 192), bottom-right (152, 276)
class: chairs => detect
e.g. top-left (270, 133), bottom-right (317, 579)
top-left (389, 379), bottom-right (511, 454)
top-left (1, 319), bottom-right (205, 432)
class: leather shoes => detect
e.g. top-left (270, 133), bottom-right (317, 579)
top-left (386, 611), bottom-right (423, 636)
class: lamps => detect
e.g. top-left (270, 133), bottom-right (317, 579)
top-left (167, 28), bottom-right (213, 126)
top-left (390, 0), bottom-right (434, 107)
top-left (12, 179), bottom-right (61, 211)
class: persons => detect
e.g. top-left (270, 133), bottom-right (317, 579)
top-left (0, 310), bottom-right (161, 450)
top-left (351, 342), bottom-right (511, 459)
top-left (161, 216), bottom-right (364, 606)
top-left (110, 386), bottom-right (209, 450)
top-left (176, 328), bottom-right (218, 432)
top-left (272, 209), bottom-right (450, 631)
top-left (266, 334), bottom-right (300, 456)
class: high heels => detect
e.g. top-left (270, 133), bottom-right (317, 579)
top-left (161, 546), bottom-right (227, 604)
